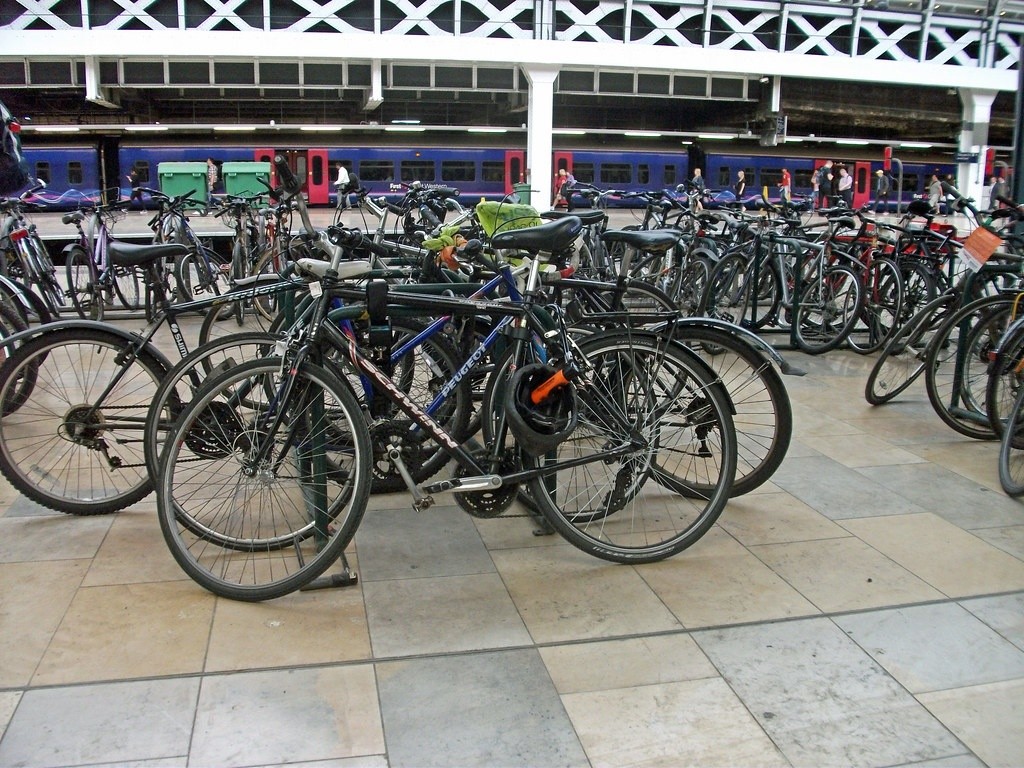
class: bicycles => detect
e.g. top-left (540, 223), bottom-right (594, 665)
top-left (620, 181), bottom-right (1024, 496)
top-left (0, 154), bottom-right (808, 602)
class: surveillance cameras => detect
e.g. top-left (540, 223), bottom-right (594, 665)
top-left (948, 90), bottom-right (957, 95)
top-left (759, 77), bottom-right (768, 83)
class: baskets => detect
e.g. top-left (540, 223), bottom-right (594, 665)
top-left (78, 188), bottom-right (127, 226)
top-left (215, 189), bottom-right (260, 229)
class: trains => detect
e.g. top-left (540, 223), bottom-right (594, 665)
top-left (0, 131), bottom-right (1012, 212)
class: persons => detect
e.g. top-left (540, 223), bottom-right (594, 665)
top-left (692, 168), bottom-right (703, 212)
top-left (121, 169), bottom-right (147, 214)
top-left (733, 170), bottom-right (746, 212)
top-left (811, 160), bottom-right (833, 208)
top-left (333, 162), bottom-right (352, 211)
top-left (778, 167), bottom-right (791, 200)
top-left (988, 176), bottom-right (1009, 211)
top-left (868, 170), bottom-right (889, 215)
top-left (928, 174), bottom-right (954, 217)
top-left (838, 169), bottom-right (852, 208)
top-left (202, 157), bottom-right (225, 215)
top-left (551, 169), bottom-right (568, 211)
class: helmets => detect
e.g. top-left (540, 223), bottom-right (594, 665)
top-left (505, 363), bottom-right (578, 457)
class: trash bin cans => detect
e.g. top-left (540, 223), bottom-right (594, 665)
top-left (222, 162), bottom-right (271, 216)
top-left (513, 183), bottom-right (530, 205)
top-left (157, 161), bottom-right (209, 216)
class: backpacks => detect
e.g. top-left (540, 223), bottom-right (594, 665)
top-left (815, 167), bottom-right (827, 183)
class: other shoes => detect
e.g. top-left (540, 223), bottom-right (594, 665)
top-left (121, 208), bottom-right (127, 213)
top-left (882, 212), bottom-right (889, 215)
top-left (868, 210), bottom-right (875, 214)
top-left (140, 210), bottom-right (148, 215)
